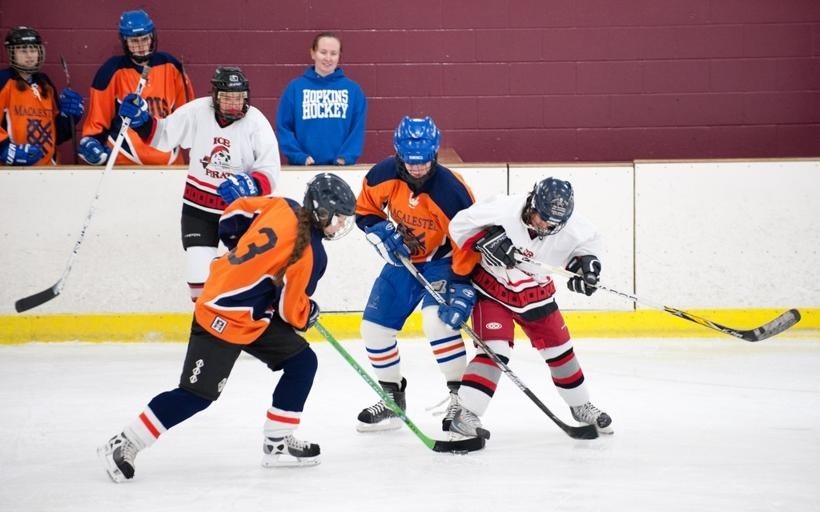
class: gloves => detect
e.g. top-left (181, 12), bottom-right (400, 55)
top-left (365, 221), bottom-right (412, 267)
top-left (56, 86), bottom-right (84, 119)
top-left (305, 296), bottom-right (320, 330)
top-left (437, 282), bottom-right (477, 330)
top-left (118, 93), bottom-right (150, 128)
top-left (472, 223), bottom-right (518, 268)
top-left (0, 136), bottom-right (43, 166)
top-left (77, 136), bottom-right (109, 166)
top-left (565, 254), bottom-right (602, 298)
top-left (216, 171), bottom-right (259, 205)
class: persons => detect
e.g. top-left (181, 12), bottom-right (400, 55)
top-left (352, 115), bottom-right (477, 434)
top-left (108, 171), bottom-right (356, 479)
top-left (0, 25), bottom-right (87, 167)
top-left (76, 9), bottom-right (194, 166)
top-left (276, 32), bottom-right (367, 165)
top-left (447, 177), bottom-right (612, 440)
top-left (119, 66), bottom-right (281, 312)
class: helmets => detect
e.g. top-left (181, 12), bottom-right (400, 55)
top-left (116, 8), bottom-right (158, 64)
top-left (390, 114), bottom-right (442, 184)
top-left (519, 176), bottom-right (577, 238)
top-left (208, 63), bottom-right (251, 123)
top-left (2, 24), bottom-right (45, 74)
top-left (301, 171), bottom-right (358, 243)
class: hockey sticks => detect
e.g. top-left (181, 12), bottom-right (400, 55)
top-left (312, 320), bottom-right (484, 451)
top-left (16, 65), bottom-right (153, 315)
top-left (395, 252), bottom-right (599, 438)
top-left (514, 253), bottom-right (800, 343)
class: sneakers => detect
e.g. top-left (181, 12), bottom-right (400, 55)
top-left (568, 401), bottom-right (613, 429)
top-left (111, 430), bottom-right (138, 480)
top-left (440, 379), bottom-right (494, 441)
top-left (358, 377), bottom-right (408, 424)
top-left (259, 435), bottom-right (322, 459)
